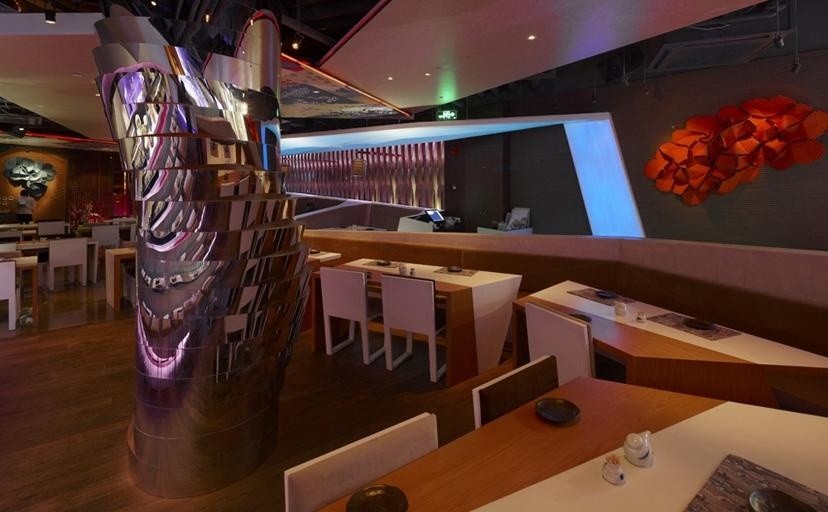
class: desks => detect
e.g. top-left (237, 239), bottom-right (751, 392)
top-left (318, 376), bottom-right (827, 511)
top-left (511, 279), bottom-right (828, 415)
top-left (311, 256), bottom-right (524, 387)
top-left (306, 247), bottom-right (340, 265)
top-left (105, 247), bottom-right (136, 313)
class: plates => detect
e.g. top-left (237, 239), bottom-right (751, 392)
top-left (535, 396), bottom-right (581, 425)
top-left (684, 318), bottom-right (713, 330)
top-left (309, 251), bottom-right (321, 254)
top-left (447, 266), bottom-right (462, 272)
top-left (365, 228), bottom-right (375, 231)
top-left (748, 488), bottom-right (818, 512)
top-left (597, 291), bottom-right (617, 298)
top-left (348, 484), bottom-right (407, 511)
top-left (376, 261), bottom-right (392, 265)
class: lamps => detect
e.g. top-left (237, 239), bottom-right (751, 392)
top-left (45, 9), bottom-right (56, 24)
top-left (290, 34), bottom-right (304, 50)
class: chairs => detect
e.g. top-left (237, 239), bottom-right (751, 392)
top-left (283, 412), bottom-right (443, 511)
top-left (318, 267), bottom-right (385, 365)
top-left (379, 272), bottom-right (446, 383)
top-left (468, 352), bottom-right (561, 430)
top-left (1, 221), bottom-right (119, 331)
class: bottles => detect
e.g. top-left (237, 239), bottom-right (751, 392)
top-left (398, 263), bottom-right (417, 276)
top-left (604, 430), bottom-right (652, 485)
top-left (615, 302), bottom-right (648, 323)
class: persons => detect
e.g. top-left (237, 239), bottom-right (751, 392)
top-left (14, 189), bottom-right (34, 224)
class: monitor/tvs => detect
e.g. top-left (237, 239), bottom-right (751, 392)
top-left (424, 209), bottom-right (446, 224)
